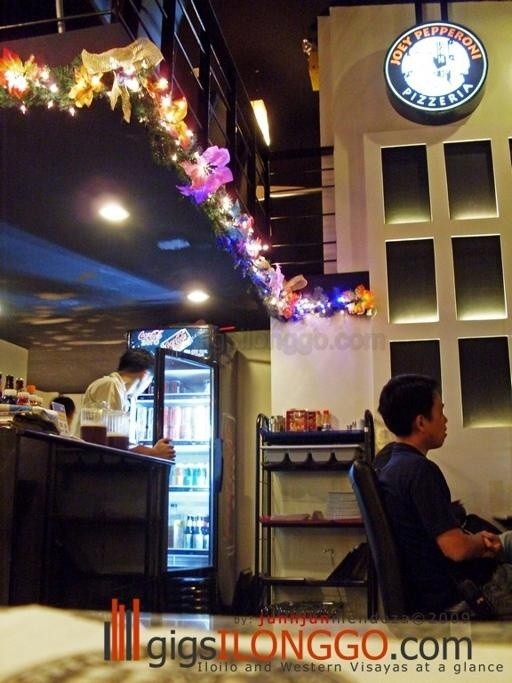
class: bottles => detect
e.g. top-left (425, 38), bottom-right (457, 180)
top-left (132, 376), bottom-right (210, 551)
top-left (166, 501), bottom-right (210, 549)
top-left (0, 373), bottom-right (39, 406)
top-left (269, 409), bottom-right (331, 434)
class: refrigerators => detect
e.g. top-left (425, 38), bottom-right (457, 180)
top-left (124, 322), bottom-right (240, 614)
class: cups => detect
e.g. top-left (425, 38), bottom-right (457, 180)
top-left (80, 407), bottom-right (108, 445)
top-left (105, 409), bottom-right (130, 450)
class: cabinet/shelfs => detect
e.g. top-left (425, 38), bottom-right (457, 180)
top-left (0, 433), bottom-right (170, 612)
top-left (254, 407), bottom-right (379, 619)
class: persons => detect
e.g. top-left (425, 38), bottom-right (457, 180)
top-left (369, 371), bottom-right (511, 623)
top-left (71, 344), bottom-right (177, 461)
top-left (48, 392), bottom-right (75, 428)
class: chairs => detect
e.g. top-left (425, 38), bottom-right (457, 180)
top-left (347, 460), bottom-right (413, 618)
